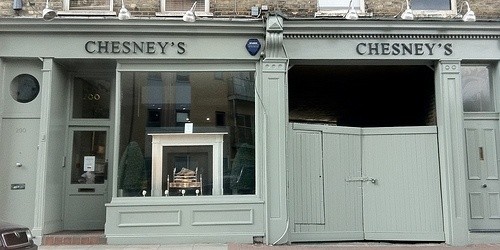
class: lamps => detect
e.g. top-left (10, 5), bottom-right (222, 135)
top-left (451, 1), bottom-right (476, 22)
top-left (119, 0), bottom-right (130, 21)
top-left (394, 0), bottom-right (416, 20)
top-left (343, 0), bottom-right (358, 20)
top-left (42, 0), bottom-right (56, 21)
top-left (182, 1), bottom-right (198, 22)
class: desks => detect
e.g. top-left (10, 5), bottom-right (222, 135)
top-left (147, 132), bottom-right (228, 195)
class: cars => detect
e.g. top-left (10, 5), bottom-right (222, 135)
top-left (0, 217), bottom-right (39, 250)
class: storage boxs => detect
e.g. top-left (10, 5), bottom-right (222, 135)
top-left (185, 123), bottom-right (193, 133)
